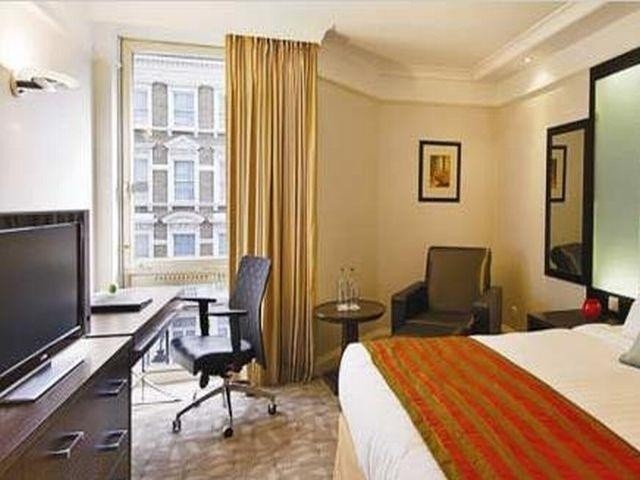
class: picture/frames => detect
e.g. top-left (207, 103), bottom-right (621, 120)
top-left (548, 145), bottom-right (567, 201)
top-left (419, 140), bottom-right (462, 201)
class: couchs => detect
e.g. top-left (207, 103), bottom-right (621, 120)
top-left (551, 243), bottom-right (584, 276)
top-left (392, 246), bottom-right (503, 336)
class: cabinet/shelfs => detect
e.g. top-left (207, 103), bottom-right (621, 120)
top-left (0, 335), bottom-right (130, 478)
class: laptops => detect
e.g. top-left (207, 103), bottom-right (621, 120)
top-left (89, 296), bottom-right (153, 313)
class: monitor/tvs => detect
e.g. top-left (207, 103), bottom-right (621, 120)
top-left (0, 209), bottom-right (91, 402)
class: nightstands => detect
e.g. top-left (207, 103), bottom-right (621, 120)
top-left (526, 308), bottom-right (620, 332)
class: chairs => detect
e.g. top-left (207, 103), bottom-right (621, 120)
top-left (170, 256), bottom-right (277, 438)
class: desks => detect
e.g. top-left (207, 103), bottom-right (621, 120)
top-left (315, 300), bottom-right (386, 396)
top-left (82, 284), bottom-right (186, 370)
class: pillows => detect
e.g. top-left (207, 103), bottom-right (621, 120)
top-left (619, 331), bottom-right (640, 369)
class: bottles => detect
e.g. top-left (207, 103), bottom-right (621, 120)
top-left (336, 268), bottom-right (349, 312)
top-left (346, 268), bottom-right (361, 311)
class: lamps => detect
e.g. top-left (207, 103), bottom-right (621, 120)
top-left (11, 58), bottom-right (81, 100)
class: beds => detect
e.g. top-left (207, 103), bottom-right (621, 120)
top-left (331, 299), bottom-right (640, 480)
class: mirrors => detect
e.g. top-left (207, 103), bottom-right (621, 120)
top-left (544, 118), bottom-right (585, 285)
